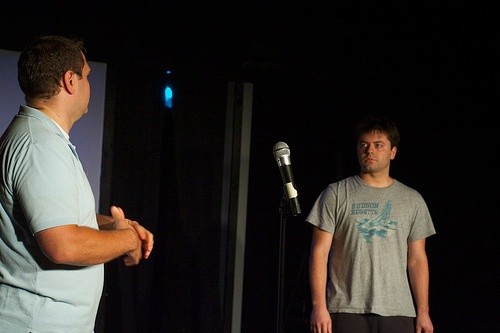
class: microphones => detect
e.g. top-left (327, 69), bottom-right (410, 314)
top-left (273, 142), bottom-right (301, 216)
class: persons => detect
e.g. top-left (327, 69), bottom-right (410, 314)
top-left (0, 35), bottom-right (154, 333)
top-left (306, 123), bottom-right (436, 333)
